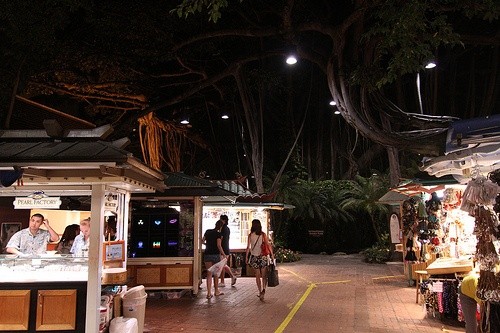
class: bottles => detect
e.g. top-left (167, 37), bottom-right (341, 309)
top-left (110, 317), bottom-right (138, 333)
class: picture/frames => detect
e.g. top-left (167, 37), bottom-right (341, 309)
top-left (103, 239), bottom-right (125, 262)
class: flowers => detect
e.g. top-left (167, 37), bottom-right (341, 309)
top-left (232, 252), bottom-right (244, 267)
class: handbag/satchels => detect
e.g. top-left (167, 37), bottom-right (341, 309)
top-left (260, 233), bottom-right (270, 256)
top-left (247, 254), bottom-right (253, 268)
top-left (267, 264), bottom-right (279, 287)
top-left (207, 258), bottom-right (228, 277)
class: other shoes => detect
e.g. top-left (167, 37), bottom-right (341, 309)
top-left (260, 289), bottom-right (265, 300)
top-left (257, 292), bottom-right (262, 297)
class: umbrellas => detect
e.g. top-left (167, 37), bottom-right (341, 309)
top-left (205, 257), bottom-right (229, 279)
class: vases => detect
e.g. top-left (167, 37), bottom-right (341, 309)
top-left (246, 265), bottom-right (260, 276)
top-left (228, 266), bottom-right (242, 277)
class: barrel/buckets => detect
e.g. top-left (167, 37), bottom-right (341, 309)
top-left (122, 293), bottom-right (147, 333)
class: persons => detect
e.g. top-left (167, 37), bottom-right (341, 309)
top-left (200, 220), bottom-right (228, 298)
top-left (216, 216), bottom-right (237, 287)
top-left (246, 219), bottom-right (275, 301)
top-left (460, 264), bottom-right (500, 333)
top-left (6, 213), bottom-right (117, 257)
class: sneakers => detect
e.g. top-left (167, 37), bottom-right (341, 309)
top-left (231, 276), bottom-right (236, 285)
top-left (218, 282), bottom-right (225, 286)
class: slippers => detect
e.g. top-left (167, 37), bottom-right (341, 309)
top-left (214, 291), bottom-right (221, 296)
top-left (206, 293), bottom-right (213, 298)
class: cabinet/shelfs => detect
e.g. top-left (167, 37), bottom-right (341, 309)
top-left (240, 210), bottom-right (260, 245)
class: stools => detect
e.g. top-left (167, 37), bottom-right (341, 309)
top-left (415, 269), bottom-right (431, 307)
